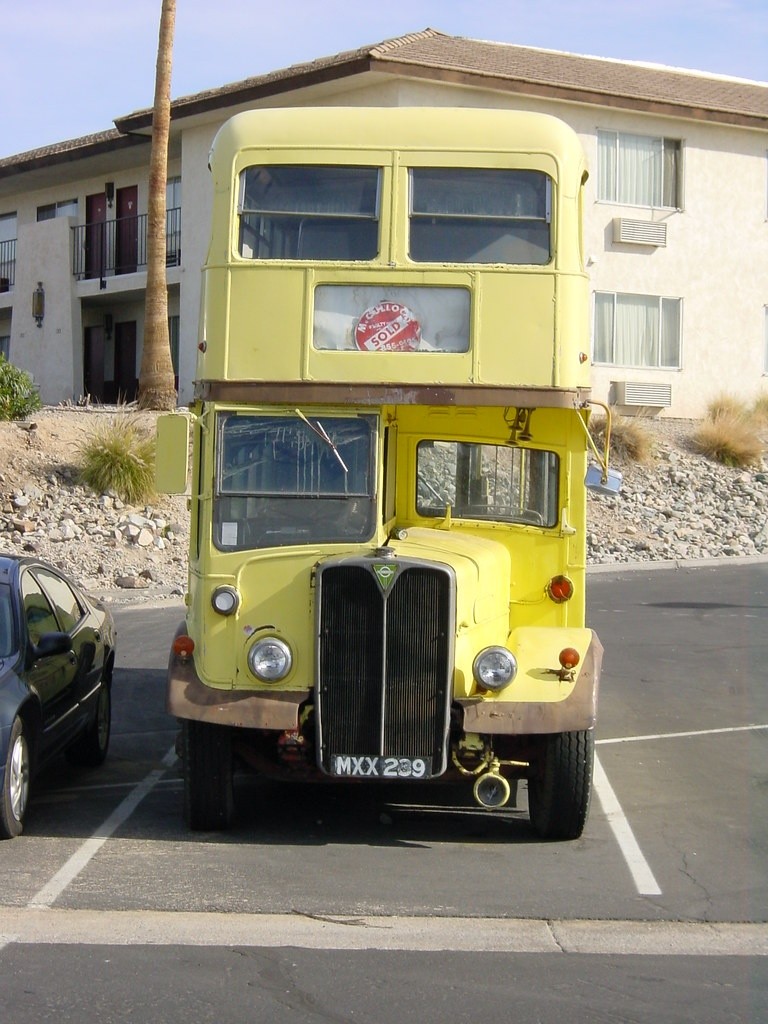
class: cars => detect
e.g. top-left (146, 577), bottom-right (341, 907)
top-left (0, 552), bottom-right (117, 840)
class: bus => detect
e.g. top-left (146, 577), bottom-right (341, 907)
top-left (154, 104), bottom-right (627, 844)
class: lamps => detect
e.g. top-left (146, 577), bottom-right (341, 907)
top-left (32, 281), bottom-right (44, 328)
top-left (104, 183), bottom-right (113, 209)
top-left (105, 314), bottom-right (112, 339)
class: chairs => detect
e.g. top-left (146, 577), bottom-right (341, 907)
top-left (297, 217), bottom-right (354, 260)
